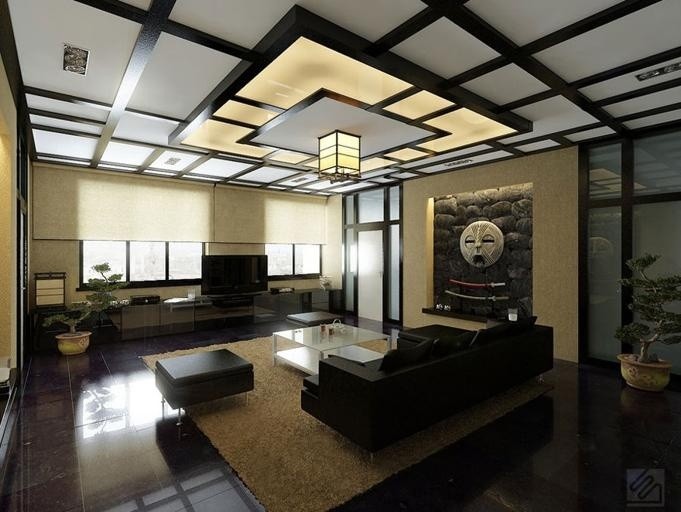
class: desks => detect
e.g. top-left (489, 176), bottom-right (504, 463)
top-left (29, 305), bottom-right (125, 355)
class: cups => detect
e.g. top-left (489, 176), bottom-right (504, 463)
top-left (320, 323), bottom-right (335, 336)
top-left (187, 288), bottom-right (195, 300)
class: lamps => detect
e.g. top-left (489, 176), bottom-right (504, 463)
top-left (166, 3), bottom-right (534, 184)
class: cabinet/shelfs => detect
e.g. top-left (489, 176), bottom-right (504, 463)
top-left (311, 291), bottom-right (330, 312)
top-left (121, 304), bottom-right (195, 341)
top-left (254, 294), bottom-right (302, 323)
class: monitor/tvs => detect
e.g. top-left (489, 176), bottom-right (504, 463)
top-left (201, 254), bottom-right (269, 298)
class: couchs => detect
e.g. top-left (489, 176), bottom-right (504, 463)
top-left (301, 315), bottom-right (554, 464)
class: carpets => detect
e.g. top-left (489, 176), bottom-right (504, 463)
top-left (139, 321), bottom-right (552, 512)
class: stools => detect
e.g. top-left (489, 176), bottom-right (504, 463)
top-left (153, 349), bottom-right (255, 426)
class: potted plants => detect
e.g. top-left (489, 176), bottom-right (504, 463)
top-left (42, 262), bottom-right (131, 356)
top-left (614, 252), bottom-right (681, 391)
